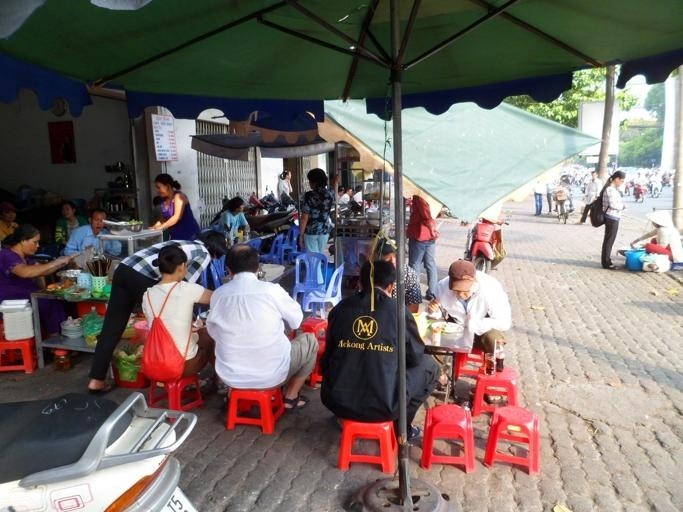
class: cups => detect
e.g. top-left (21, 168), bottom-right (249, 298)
top-left (429, 323), bottom-right (441, 346)
top-left (484, 353), bottom-right (495, 376)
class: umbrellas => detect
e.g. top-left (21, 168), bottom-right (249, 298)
top-left (190, 130), bottom-right (335, 199)
top-left (0, 0), bottom-right (683, 512)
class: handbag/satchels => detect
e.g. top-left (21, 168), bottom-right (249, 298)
top-left (640, 254), bottom-right (671, 274)
top-left (624, 250), bottom-right (648, 272)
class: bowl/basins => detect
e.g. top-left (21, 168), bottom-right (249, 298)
top-left (105, 221), bottom-right (145, 236)
top-left (57, 269), bottom-right (83, 283)
top-left (347, 218), bottom-right (367, 226)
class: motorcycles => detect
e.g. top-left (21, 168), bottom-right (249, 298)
top-left (209, 191), bottom-right (353, 235)
top-left (575, 155), bottom-right (672, 202)
top-left (465, 211), bottom-right (510, 274)
top-left (0, 392), bottom-right (197, 510)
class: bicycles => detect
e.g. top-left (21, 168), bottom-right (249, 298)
top-left (551, 184), bottom-right (575, 223)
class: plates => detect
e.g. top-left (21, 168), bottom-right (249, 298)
top-left (1, 299), bottom-right (34, 341)
top-left (431, 321), bottom-right (465, 336)
top-left (60, 321), bottom-right (83, 339)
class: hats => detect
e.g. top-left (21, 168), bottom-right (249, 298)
top-left (647, 210), bottom-right (673, 228)
top-left (448, 258), bottom-right (477, 291)
top-left (376, 237), bottom-right (398, 256)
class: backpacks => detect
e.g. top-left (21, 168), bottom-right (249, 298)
top-left (142, 282), bottom-right (192, 383)
top-left (591, 182), bottom-right (610, 227)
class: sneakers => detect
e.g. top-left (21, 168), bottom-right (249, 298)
top-left (187, 378), bottom-right (211, 391)
top-left (396, 425), bottom-right (421, 441)
top-left (216, 382), bottom-right (229, 395)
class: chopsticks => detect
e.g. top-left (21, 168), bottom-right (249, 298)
top-left (86, 257), bottom-right (112, 276)
top-left (432, 293), bottom-right (455, 321)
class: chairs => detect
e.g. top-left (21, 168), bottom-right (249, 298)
top-left (193, 225), bottom-right (346, 309)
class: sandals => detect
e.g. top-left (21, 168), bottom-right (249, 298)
top-left (283, 395), bottom-right (311, 411)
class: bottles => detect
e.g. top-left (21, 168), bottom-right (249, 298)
top-left (494, 339), bottom-right (506, 373)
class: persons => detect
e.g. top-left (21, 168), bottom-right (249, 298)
top-left (318, 259), bottom-right (441, 445)
top-left (338, 182), bottom-right (378, 214)
top-left (278, 169), bottom-right (293, 205)
top-left (64, 208), bottom-right (123, 258)
top-left (630, 212), bottom-right (683, 270)
top-left (0, 225), bottom-right (75, 321)
top-left (150, 195), bottom-right (170, 241)
top-left (147, 173), bottom-right (200, 240)
top-left (373, 237), bottom-right (422, 313)
top-left (404, 187), bottom-right (438, 301)
top-left (54, 201), bottom-right (89, 258)
top-left (299, 167), bottom-right (341, 286)
top-left (600, 170), bottom-right (627, 270)
top-left (141, 244), bottom-right (214, 389)
top-left (425, 259), bottom-right (514, 370)
top-left (533, 169), bottom-right (601, 225)
top-left (210, 196), bottom-right (251, 251)
top-left (627, 168), bottom-right (676, 203)
top-left (0, 201), bottom-right (19, 241)
top-left (87, 229), bottom-right (229, 396)
top-left (205, 244), bottom-right (329, 410)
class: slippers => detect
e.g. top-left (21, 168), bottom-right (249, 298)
top-left (89, 384), bottom-right (112, 395)
